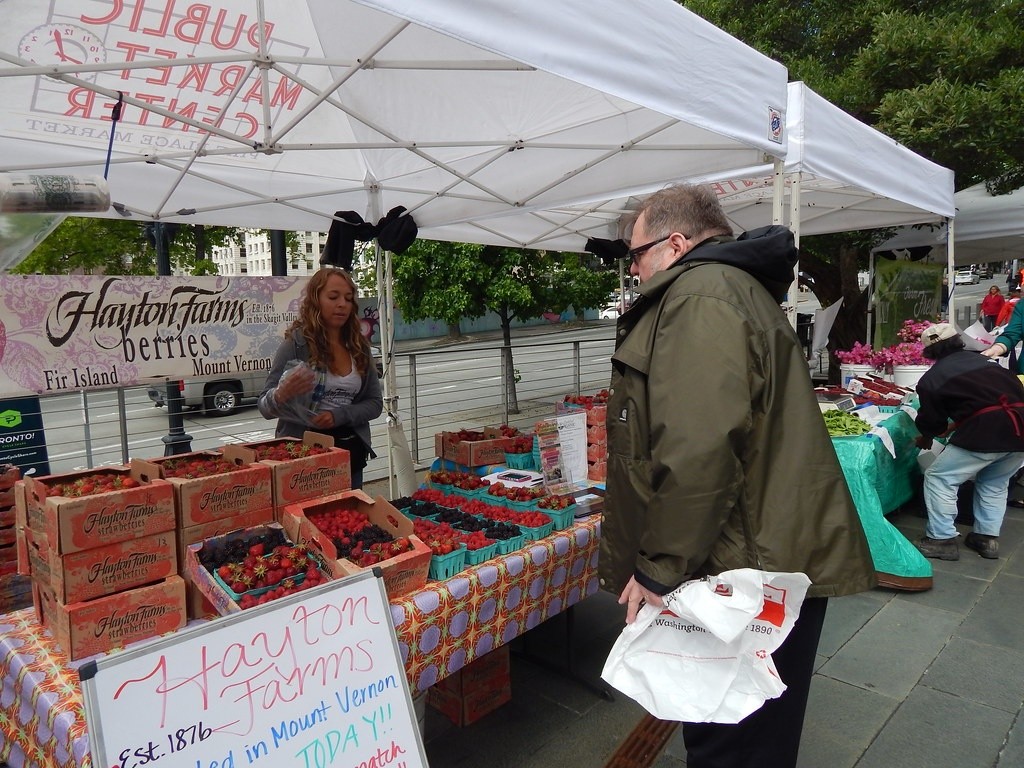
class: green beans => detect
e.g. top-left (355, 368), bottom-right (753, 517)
top-left (822, 408), bottom-right (871, 437)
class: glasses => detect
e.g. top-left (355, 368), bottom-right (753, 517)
top-left (628, 233), bottom-right (692, 266)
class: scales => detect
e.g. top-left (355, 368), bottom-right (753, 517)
top-left (816, 393), bottom-right (858, 414)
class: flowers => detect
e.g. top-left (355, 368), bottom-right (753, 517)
top-left (834, 317), bottom-right (960, 374)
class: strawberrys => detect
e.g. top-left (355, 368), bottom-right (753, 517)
top-left (218, 543), bottom-right (327, 610)
top-left (564, 389), bottom-right (608, 409)
top-left (157, 454), bottom-right (244, 478)
top-left (449, 427), bottom-right (486, 441)
top-left (40, 470), bottom-right (142, 498)
top-left (349, 536), bottom-right (409, 568)
top-left (499, 424), bottom-right (533, 454)
top-left (429, 470), bottom-right (576, 511)
top-left (252, 440), bottom-right (331, 461)
top-left (423, 536), bottom-right (460, 554)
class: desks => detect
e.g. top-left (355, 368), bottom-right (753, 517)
top-left (0, 514), bottom-right (607, 768)
top-left (817, 398), bottom-right (935, 592)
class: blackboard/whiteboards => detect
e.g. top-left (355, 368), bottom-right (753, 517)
top-left (78, 566), bottom-right (430, 768)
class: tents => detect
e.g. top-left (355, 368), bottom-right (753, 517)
top-left (0, 0), bottom-right (954, 497)
top-left (867, 181), bottom-right (1024, 348)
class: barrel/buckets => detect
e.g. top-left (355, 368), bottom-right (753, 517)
top-left (917, 473), bottom-right (976, 526)
top-left (893, 363), bottom-right (934, 390)
top-left (840, 363), bottom-right (884, 389)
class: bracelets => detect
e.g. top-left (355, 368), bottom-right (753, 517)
top-left (994, 344), bottom-right (1005, 354)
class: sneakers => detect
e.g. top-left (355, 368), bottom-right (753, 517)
top-left (966, 531), bottom-right (999, 559)
top-left (911, 536), bottom-right (959, 560)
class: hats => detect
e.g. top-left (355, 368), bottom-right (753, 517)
top-left (921, 323), bottom-right (957, 347)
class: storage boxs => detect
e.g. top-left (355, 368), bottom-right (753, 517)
top-left (814, 373), bottom-right (919, 414)
top-left (412, 645), bottom-right (512, 744)
top-left (0, 390), bottom-right (610, 663)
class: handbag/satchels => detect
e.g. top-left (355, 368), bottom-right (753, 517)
top-left (994, 334), bottom-right (1024, 375)
top-left (601, 569), bottom-right (812, 723)
top-left (979, 310), bottom-right (985, 325)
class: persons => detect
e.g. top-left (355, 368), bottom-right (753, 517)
top-left (914, 323), bottom-right (1024, 558)
top-left (981, 285), bottom-right (1005, 333)
top-left (942, 279), bottom-right (949, 312)
top-left (825, 280), bottom-right (875, 384)
top-left (257, 267), bottom-right (382, 491)
top-left (980, 293), bottom-right (1024, 376)
top-left (599, 186), bottom-right (879, 767)
top-left (1006, 269), bottom-right (1021, 294)
